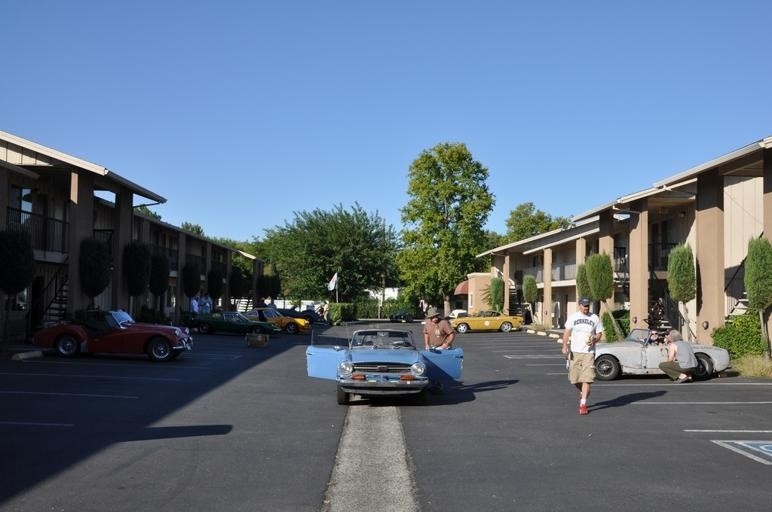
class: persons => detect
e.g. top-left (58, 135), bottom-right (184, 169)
top-left (318, 307), bottom-right (324, 321)
top-left (659, 330), bottom-right (699, 383)
top-left (423, 307), bottom-right (456, 393)
top-left (257, 297), bottom-right (267, 308)
top-left (562, 297), bottom-right (602, 415)
top-left (192, 293), bottom-right (213, 313)
top-left (328, 272), bottom-right (337, 291)
top-left (267, 299), bottom-right (276, 308)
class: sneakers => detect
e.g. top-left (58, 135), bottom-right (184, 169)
top-left (673, 377), bottom-right (687, 384)
top-left (578, 403), bottom-right (590, 415)
top-left (427, 381), bottom-right (444, 395)
top-left (684, 376), bottom-right (694, 381)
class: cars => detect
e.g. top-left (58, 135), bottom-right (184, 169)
top-left (306, 320), bottom-right (464, 406)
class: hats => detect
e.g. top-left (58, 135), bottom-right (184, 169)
top-left (426, 308), bottom-right (441, 318)
top-left (577, 298), bottom-right (590, 306)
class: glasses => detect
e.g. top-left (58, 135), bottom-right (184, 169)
top-left (650, 333), bottom-right (658, 335)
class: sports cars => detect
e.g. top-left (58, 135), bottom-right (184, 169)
top-left (449, 309), bottom-right (523, 333)
top-left (31, 310), bottom-right (193, 362)
top-left (191, 308), bottom-right (313, 337)
top-left (594, 329), bottom-right (729, 381)
